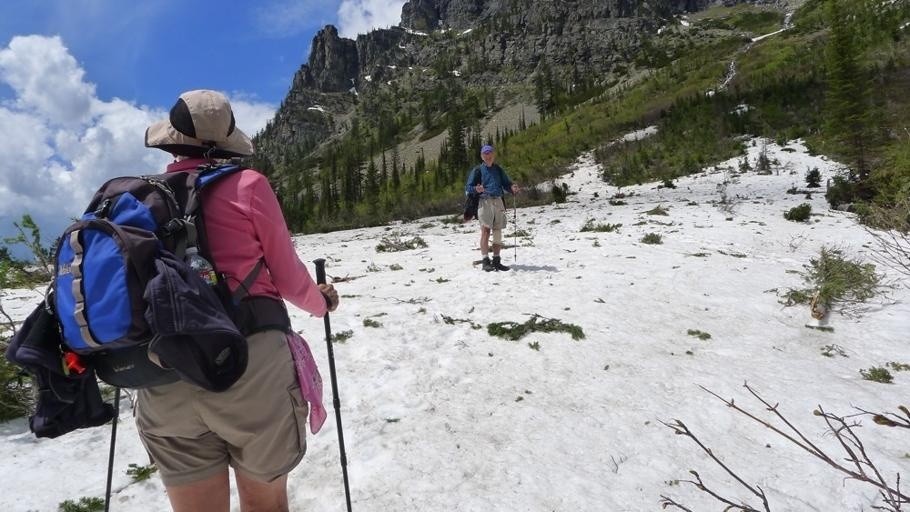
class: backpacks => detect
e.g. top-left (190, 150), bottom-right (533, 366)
top-left (49, 159), bottom-right (296, 395)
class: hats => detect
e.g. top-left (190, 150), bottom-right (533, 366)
top-left (480, 145), bottom-right (495, 154)
top-left (143, 88), bottom-right (255, 158)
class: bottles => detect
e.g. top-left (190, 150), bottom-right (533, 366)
top-left (185, 246), bottom-right (220, 289)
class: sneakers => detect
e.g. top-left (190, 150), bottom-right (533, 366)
top-left (482, 258), bottom-right (494, 272)
top-left (492, 256), bottom-right (509, 271)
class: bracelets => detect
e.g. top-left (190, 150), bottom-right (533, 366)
top-left (319, 291), bottom-right (333, 312)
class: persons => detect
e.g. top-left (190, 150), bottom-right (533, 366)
top-left (133, 85), bottom-right (341, 512)
top-left (465, 145), bottom-right (520, 272)
